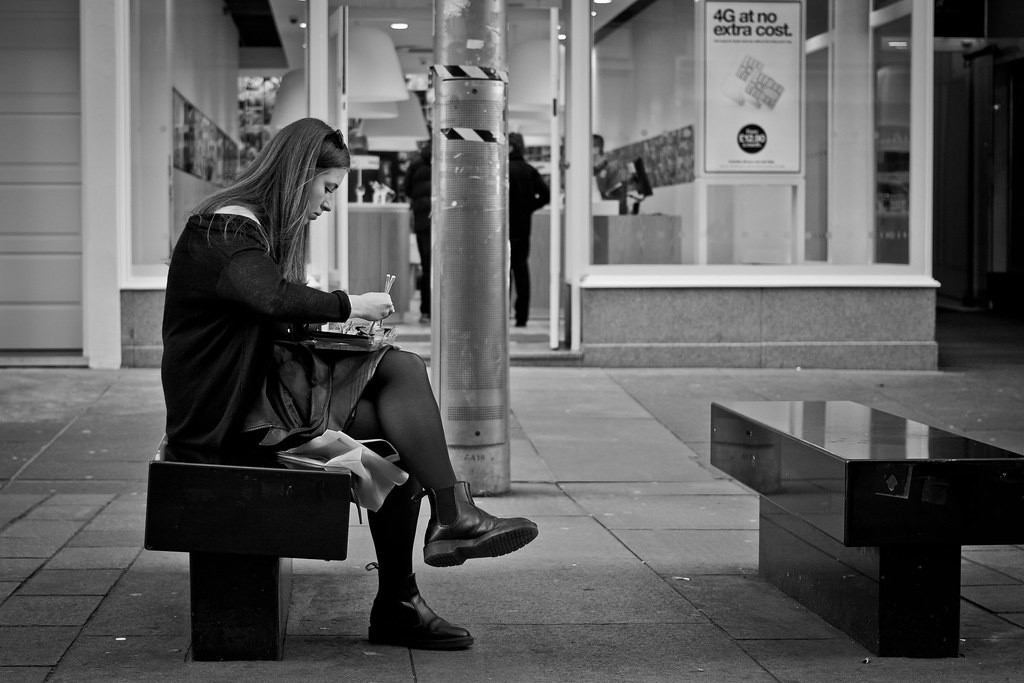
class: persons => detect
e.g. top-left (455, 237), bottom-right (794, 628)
top-left (161, 118), bottom-right (538, 650)
top-left (402, 139), bottom-right (432, 323)
top-left (593, 135), bottom-right (623, 201)
top-left (348, 135), bottom-right (386, 204)
top-left (509, 132), bottom-right (550, 327)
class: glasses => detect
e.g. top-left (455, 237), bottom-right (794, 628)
top-left (322, 128), bottom-right (345, 149)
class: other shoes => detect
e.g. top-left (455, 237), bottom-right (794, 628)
top-left (419, 313), bottom-right (430, 323)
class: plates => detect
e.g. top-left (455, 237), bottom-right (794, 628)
top-left (312, 326), bottom-right (392, 347)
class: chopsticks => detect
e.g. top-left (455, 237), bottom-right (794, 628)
top-left (369, 274), bottom-right (396, 332)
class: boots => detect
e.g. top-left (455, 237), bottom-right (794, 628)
top-left (365, 562), bottom-right (474, 650)
top-left (422, 481), bottom-right (538, 569)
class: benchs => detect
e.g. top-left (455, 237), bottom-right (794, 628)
top-left (710, 401), bottom-right (1024, 660)
top-left (145, 432), bottom-right (353, 659)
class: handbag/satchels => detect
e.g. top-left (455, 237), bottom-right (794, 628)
top-left (256, 423), bottom-right (408, 514)
